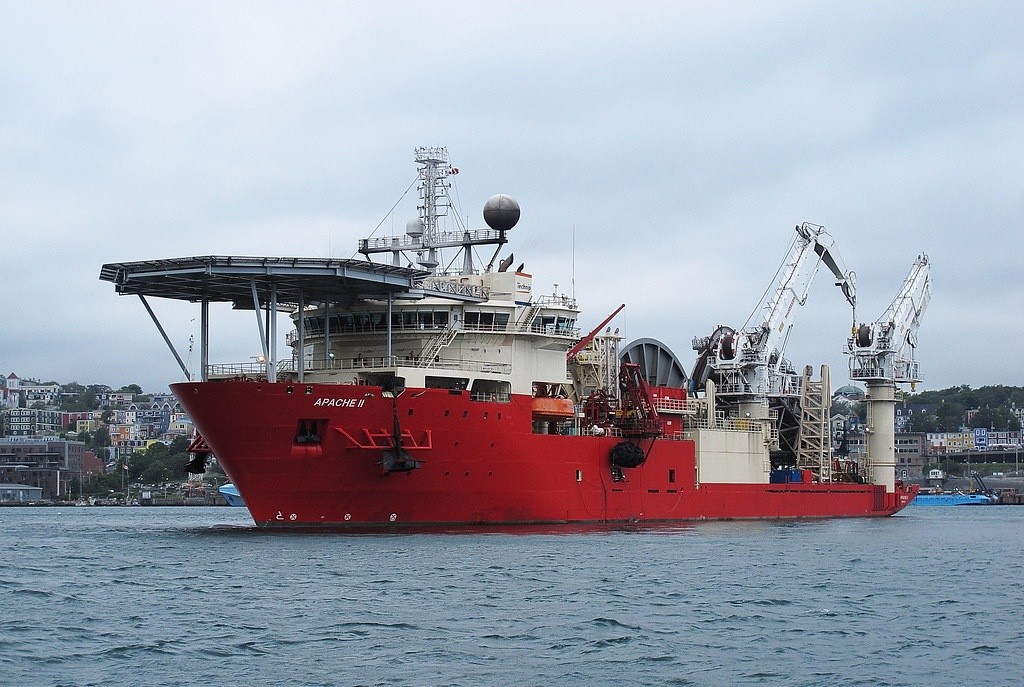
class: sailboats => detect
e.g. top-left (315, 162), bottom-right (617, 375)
top-left (100, 146), bottom-right (934, 529)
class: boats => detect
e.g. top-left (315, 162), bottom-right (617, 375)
top-left (217, 484), bottom-right (245, 507)
top-left (907, 472), bottom-right (994, 505)
